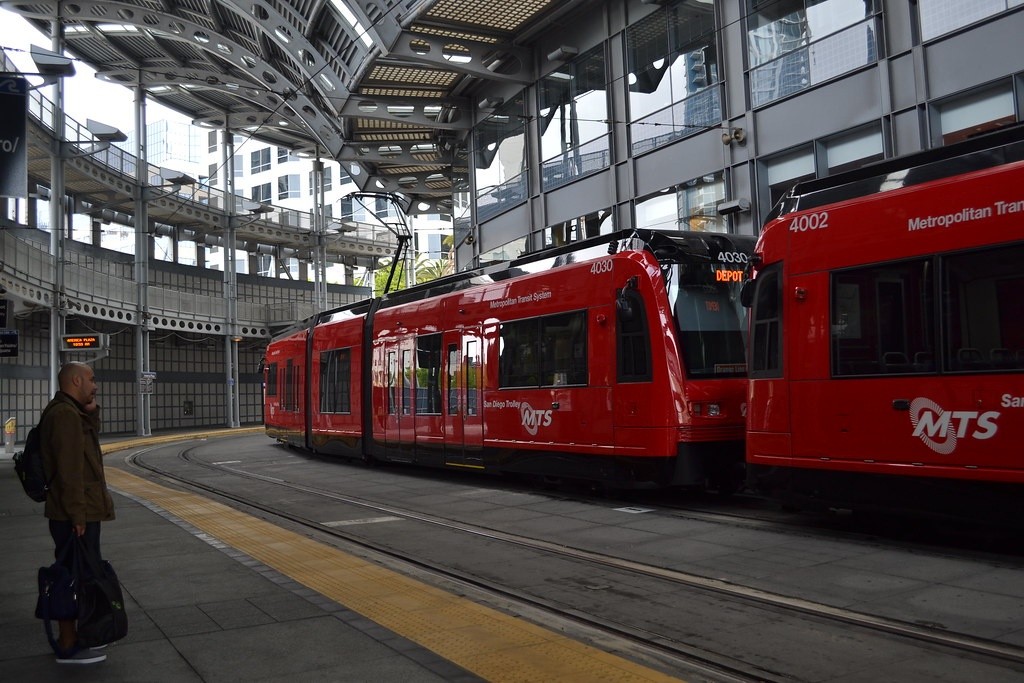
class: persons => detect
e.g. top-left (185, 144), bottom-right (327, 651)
top-left (33, 360), bottom-right (117, 663)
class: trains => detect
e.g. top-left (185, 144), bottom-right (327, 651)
top-left (261, 123), bottom-right (1024, 549)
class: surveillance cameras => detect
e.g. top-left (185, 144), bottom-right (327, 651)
top-left (60, 296), bottom-right (67, 305)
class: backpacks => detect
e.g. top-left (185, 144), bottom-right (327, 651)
top-left (12, 402), bottom-right (68, 503)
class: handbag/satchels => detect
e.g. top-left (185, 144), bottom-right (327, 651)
top-left (35, 532), bottom-right (128, 658)
